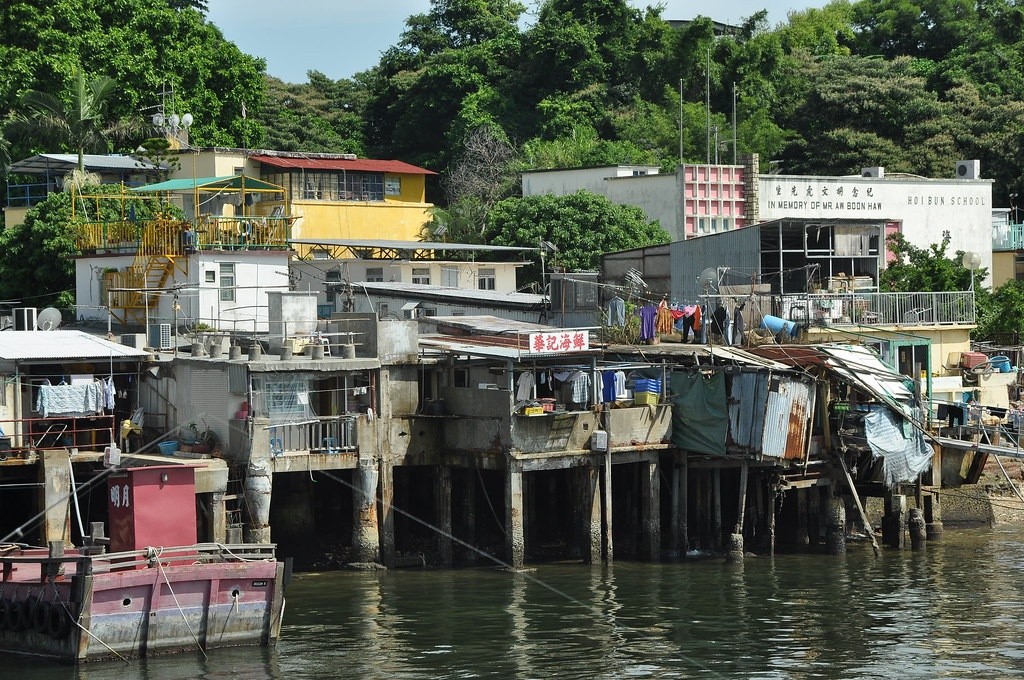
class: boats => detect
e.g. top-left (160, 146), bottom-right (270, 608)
top-left (0, 458), bottom-right (282, 662)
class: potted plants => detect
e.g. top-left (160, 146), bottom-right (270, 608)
top-left (193, 418), bottom-right (213, 453)
top-left (179, 213), bottom-right (194, 231)
top-left (180, 423), bottom-right (198, 453)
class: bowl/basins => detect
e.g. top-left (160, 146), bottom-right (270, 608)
top-left (181, 438), bottom-right (194, 445)
top-left (158, 441), bottom-right (178, 455)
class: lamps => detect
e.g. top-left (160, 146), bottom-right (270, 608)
top-left (961, 250), bottom-right (981, 292)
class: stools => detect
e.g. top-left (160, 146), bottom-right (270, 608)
top-left (316, 338), bottom-right (332, 358)
top-left (903, 306), bottom-right (933, 323)
top-left (271, 438), bottom-right (284, 456)
top-left (322, 438), bottom-right (338, 453)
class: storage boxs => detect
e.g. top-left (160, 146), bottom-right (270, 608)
top-left (990, 356), bottom-right (1011, 374)
top-left (948, 351), bottom-right (973, 365)
top-left (827, 277), bottom-right (848, 291)
top-left (849, 276), bottom-right (873, 292)
top-left (521, 401), bottom-right (566, 415)
top-left (961, 353), bottom-right (987, 368)
top-left (634, 378), bottom-right (662, 407)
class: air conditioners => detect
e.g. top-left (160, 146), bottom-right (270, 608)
top-left (148, 323), bottom-right (171, 352)
top-left (956, 159), bottom-right (980, 180)
top-left (120, 333), bottom-right (147, 351)
top-left (860, 167), bottom-right (885, 179)
top-left (12, 307), bottom-right (37, 332)
top-left (196, 331), bottom-right (232, 353)
